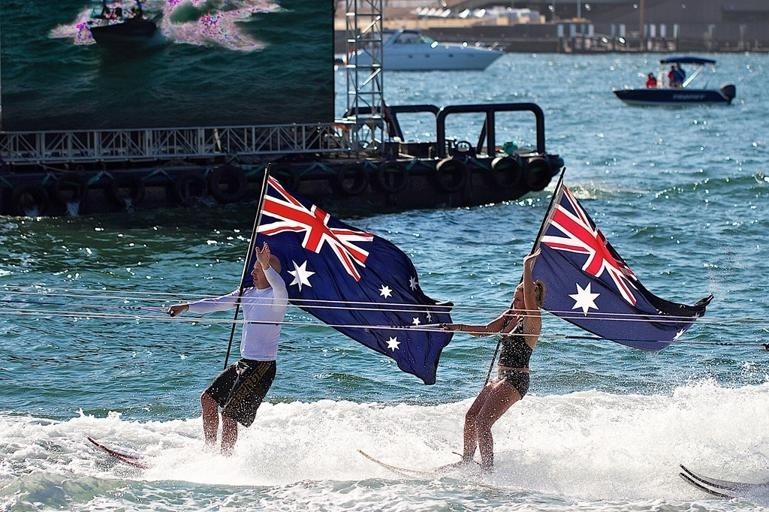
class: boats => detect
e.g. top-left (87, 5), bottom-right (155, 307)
top-left (335, 29), bottom-right (504, 71)
top-left (90, 0), bottom-right (164, 58)
top-left (613, 57), bottom-right (735, 106)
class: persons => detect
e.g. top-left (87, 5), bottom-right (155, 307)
top-left (646, 72), bottom-right (656, 89)
top-left (434, 248), bottom-right (545, 480)
top-left (668, 64), bottom-right (686, 89)
top-left (166, 241), bottom-right (288, 460)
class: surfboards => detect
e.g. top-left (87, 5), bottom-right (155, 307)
top-left (679, 463), bottom-right (768, 500)
top-left (358, 449), bottom-right (440, 480)
top-left (87, 436), bottom-right (151, 470)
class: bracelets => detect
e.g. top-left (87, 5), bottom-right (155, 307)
top-left (459, 324), bottom-right (462, 331)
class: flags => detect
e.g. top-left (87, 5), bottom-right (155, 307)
top-left (240, 174), bottom-right (454, 385)
top-left (526, 179), bottom-right (713, 352)
